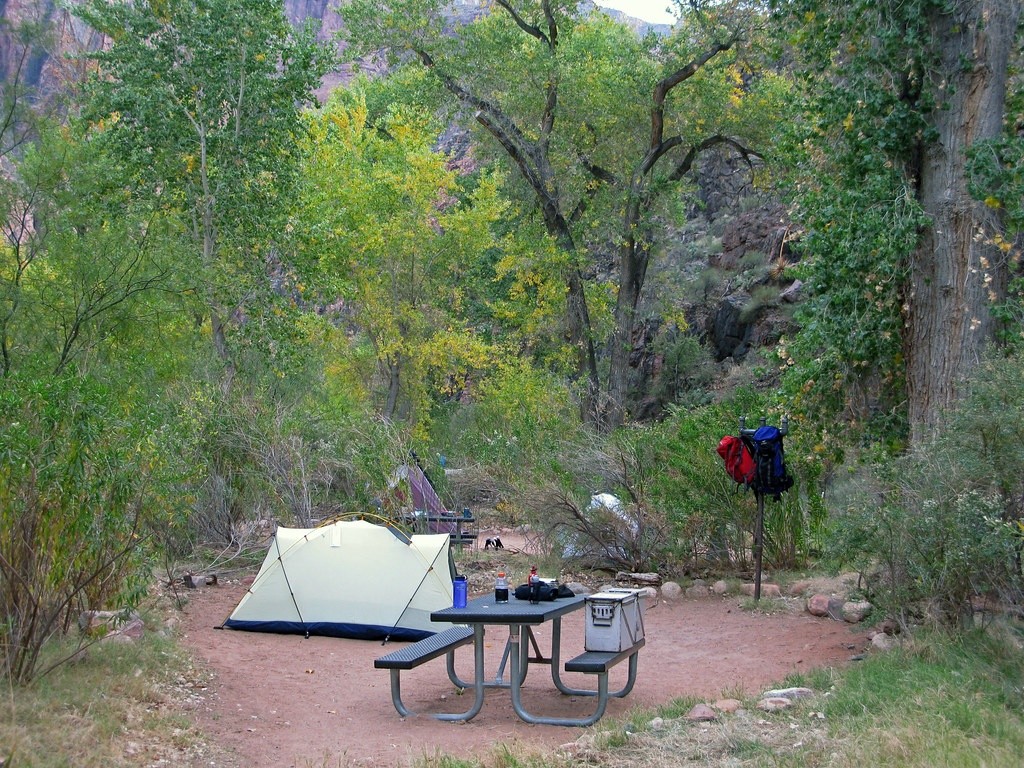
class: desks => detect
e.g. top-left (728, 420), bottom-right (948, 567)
top-left (430, 590), bottom-right (590, 724)
top-left (395, 515), bottom-right (475, 550)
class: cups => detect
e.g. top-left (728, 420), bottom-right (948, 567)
top-left (464, 507), bottom-right (470, 517)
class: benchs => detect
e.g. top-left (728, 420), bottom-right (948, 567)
top-left (450, 539), bottom-right (473, 544)
top-left (374, 626), bottom-right (485, 721)
top-left (450, 533), bottom-right (478, 539)
top-left (565, 637), bottom-right (645, 727)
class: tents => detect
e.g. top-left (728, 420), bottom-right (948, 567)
top-left (562, 493), bottom-right (639, 559)
top-left (227, 524), bottom-right (465, 641)
top-left (354, 456), bottom-right (464, 540)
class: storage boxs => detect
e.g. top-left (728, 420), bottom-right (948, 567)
top-left (584, 592), bottom-right (636, 653)
top-left (603, 588), bottom-right (647, 642)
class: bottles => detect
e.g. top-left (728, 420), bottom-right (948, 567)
top-left (528, 565), bottom-right (538, 587)
top-left (495, 573), bottom-right (509, 604)
top-left (530, 574), bottom-right (539, 604)
top-left (453, 574), bottom-right (468, 608)
